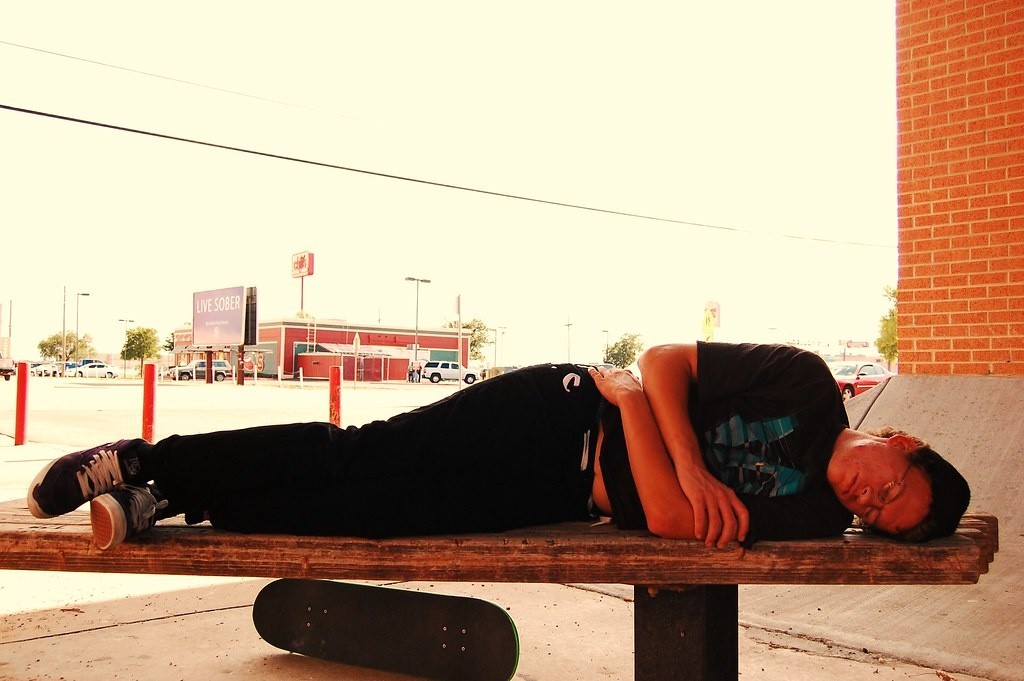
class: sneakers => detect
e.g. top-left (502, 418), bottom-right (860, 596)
top-left (27, 438), bottom-right (150, 519)
top-left (90, 484), bottom-right (169, 550)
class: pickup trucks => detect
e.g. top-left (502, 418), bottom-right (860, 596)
top-left (0, 352), bottom-right (15, 380)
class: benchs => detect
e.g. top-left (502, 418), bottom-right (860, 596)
top-left (0, 498), bottom-right (998, 680)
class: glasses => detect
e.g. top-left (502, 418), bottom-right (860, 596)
top-left (859, 463), bottom-right (912, 528)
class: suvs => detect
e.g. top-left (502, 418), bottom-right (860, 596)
top-left (170, 360), bottom-right (234, 382)
top-left (416, 358), bottom-right (478, 384)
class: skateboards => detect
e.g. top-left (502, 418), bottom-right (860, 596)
top-left (250, 578), bottom-right (520, 681)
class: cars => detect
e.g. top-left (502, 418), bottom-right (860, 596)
top-left (835, 362), bottom-right (897, 402)
top-left (66, 363), bottom-right (119, 380)
top-left (32, 358), bottom-right (103, 381)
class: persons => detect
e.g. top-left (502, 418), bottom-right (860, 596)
top-left (27, 340), bottom-right (971, 549)
top-left (408, 362), bottom-right (422, 383)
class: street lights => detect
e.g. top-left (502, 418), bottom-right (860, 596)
top-left (75, 293), bottom-right (90, 380)
top-left (118, 319), bottom-right (135, 377)
top-left (405, 276), bottom-right (432, 365)
top-left (564, 323), bottom-right (571, 363)
top-left (490, 329), bottom-right (497, 366)
top-left (602, 330), bottom-right (610, 349)
